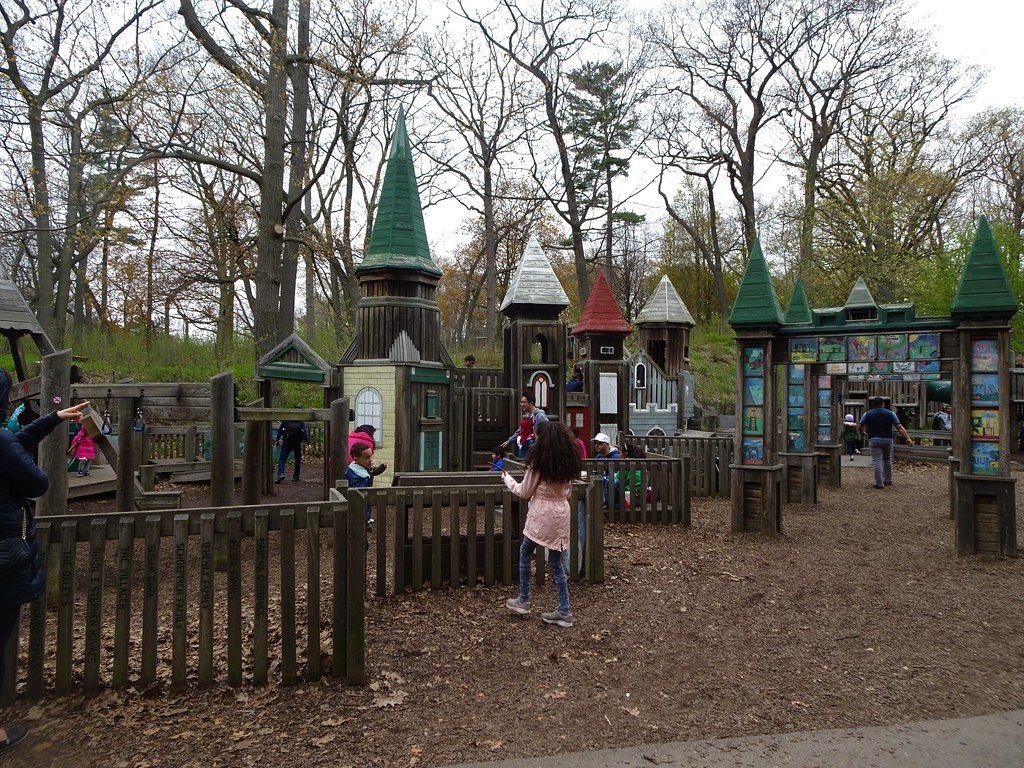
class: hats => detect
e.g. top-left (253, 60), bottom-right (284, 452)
top-left (590, 433), bottom-right (610, 445)
top-left (846, 414), bottom-right (854, 422)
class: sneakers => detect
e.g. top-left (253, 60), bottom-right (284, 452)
top-left (506, 597), bottom-right (531, 615)
top-left (541, 608), bottom-right (572, 628)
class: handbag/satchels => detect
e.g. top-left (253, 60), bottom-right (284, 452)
top-left (0, 538), bottom-right (45, 605)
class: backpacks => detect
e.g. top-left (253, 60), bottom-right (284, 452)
top-left (282, 421), bottom-right (302, 446)
top-left (603, 476), bottom-right (624, 510)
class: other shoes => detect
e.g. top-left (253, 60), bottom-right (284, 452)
top-left (274, 475), bottom-right (286, 484)
top-left (292, 477), bottom-right (302, 482)
top-left (77, 471), bottom-right (84, 477)
top-left (850, 455), bottom-right (854, 460)
top-left (885, 482), bottom-right (892, 486)
top-left (855, 449), bottom-right (861, 453)
top-left (368, 519), bottom-right (375, 524)
top-left (874, 484), bottom-right (883, 489)
top-left (84, 471), bottom-right (93, 476)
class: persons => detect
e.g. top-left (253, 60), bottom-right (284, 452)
top-left (857, 397), bottom-right (913, 489)
top-left (567, 373), bottom-right (583, 392)
top-left (932, 406), bottom-right (951, 446)
top-left (328, 424), bottom-right (376, 530)
top-left (462, 355), bottom-right (482, 418)
top-left (500, 392), bottom-right (548, 459)
top-left (343, 442), bottom-right (388, 550)
top-left (839, 414), bottom-right (861, 461)
top-left (590, 433), bottom-right (647, 509)
top-left (7, 403), bottom-right (38, 466)
top-left (274, 407), bottom-right (311, 484)
top-left (488, 446), bottom-right (504, 470)
top-left (570, 429), bottom-right (585, 458)
top-left (71, 425), bottom-right (96, 477)
top-left (0, 368), bottom-right (90, 750)
top-left (501, 421), bottom-right (581, 628)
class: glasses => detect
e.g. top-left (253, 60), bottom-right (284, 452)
top-left (520, 402), bottom-right (527, 404)
